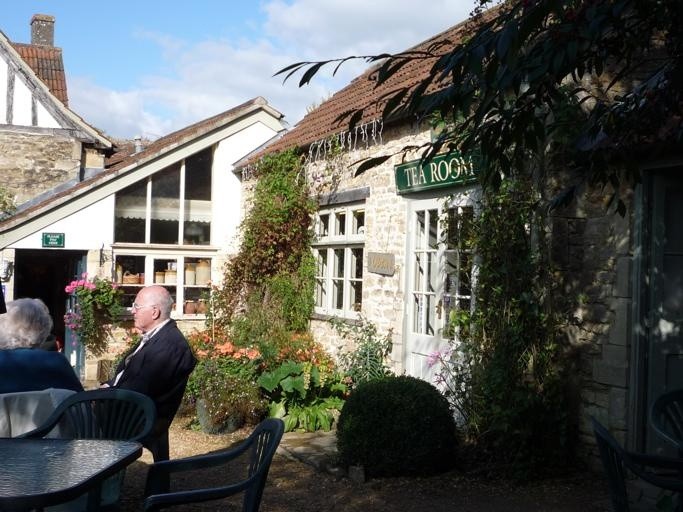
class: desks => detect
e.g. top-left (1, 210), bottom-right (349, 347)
top-left (0, 437), bottom-right (142, 510)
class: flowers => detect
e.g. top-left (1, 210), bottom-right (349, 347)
top-left (64, 271), bottom-right (123, 348)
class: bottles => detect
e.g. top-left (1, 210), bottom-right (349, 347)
top-left (185, 264), bottom-right (195, 285)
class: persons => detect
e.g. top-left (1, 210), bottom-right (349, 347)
top-left (89, 285), bottom-right (191, 442)
top-left (0, 297), bottom-right (86, 394)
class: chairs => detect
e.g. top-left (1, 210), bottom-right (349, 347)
top-left (12, 387), bottom-right (284, 511)
top-left (588, 388), bottom-right (683, 512)
